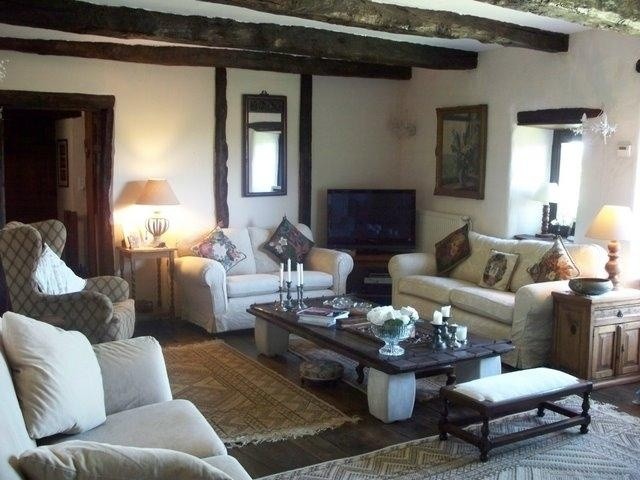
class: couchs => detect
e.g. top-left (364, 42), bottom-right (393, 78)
top-left (1, 308), bottom-right (259, 480)
top-left (387, 219), bottom-right (607, 368)
top-left (1, 218), bottom-right (137, 346)
top-left (168, 218), bottom-right (356, 337)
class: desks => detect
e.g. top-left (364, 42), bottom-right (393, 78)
top-left (116, 243), bottom-right (180, 321)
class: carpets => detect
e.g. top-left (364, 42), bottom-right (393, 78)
top-left (152, 335), bottom-right (364, 449)
top-left (246, 392), bottom-right (639, 480)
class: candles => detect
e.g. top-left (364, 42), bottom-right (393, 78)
top-left (280, 262), bottom-right (284, 287)
top-left (301, 263), bottom-right (304, 286)
top-left (287, 256), bottom-right (291, 282)
top-left (443, 304), bottom-right (451, 317)
top-left (434, 310), bottom-right (443, 326)
top-left (296, 263), bottom-right (301, 287)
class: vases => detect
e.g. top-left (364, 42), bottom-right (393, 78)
top-left (371, 325), bottom-right (417, 358)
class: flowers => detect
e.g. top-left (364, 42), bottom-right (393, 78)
top-left (364, 304), bottom-right (421, 332)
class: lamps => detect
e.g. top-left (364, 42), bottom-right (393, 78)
top-left (135, 179), bottom-right (181, 248)
top-left (586, 203), bottom-right (637, 293)
top-left (534, 181), bottom-right (562, 237)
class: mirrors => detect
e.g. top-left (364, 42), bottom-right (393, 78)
top-left (242, 90), bottom-right (288, 199)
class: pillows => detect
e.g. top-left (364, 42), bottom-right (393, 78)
top-left (433, 222), bottom-right (472, 276)
top-left (191, 225), bottom-right (247, 272)
top-left (525, 233), bottom-right (581, 284)
top-left (2, 310), bottom-right (109, 440)
top-left (8, 437), bottom-right (236, 480)
top-left (479, 247), bottom-right (521, 292)
top-left (262, 214), bottom-right (315, 269)
top-left (33, 240), bottom-right (88, 296)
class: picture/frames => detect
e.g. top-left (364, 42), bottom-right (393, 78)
top-left (432, 103), bottom-right (490, 201)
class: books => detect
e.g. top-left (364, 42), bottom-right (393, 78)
top-left (297, 306), bottom-right (334, 316)
top-left (299, 311), bottom-right (349, 324)
top-left (298, 320), bottom-right (336, 328)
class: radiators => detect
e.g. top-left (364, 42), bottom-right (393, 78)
top-left (416, 208), bottom-right (468, 252)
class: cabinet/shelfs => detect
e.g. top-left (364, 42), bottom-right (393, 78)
top-left (550, 288), bottom-right (640, 389)
top-left (345, 253), bottom-right (393, 300)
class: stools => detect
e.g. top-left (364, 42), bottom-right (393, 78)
top-left (295, 357), bottom-right (346, 390)
top-left (437, 366), bottom-right (595, 461)
